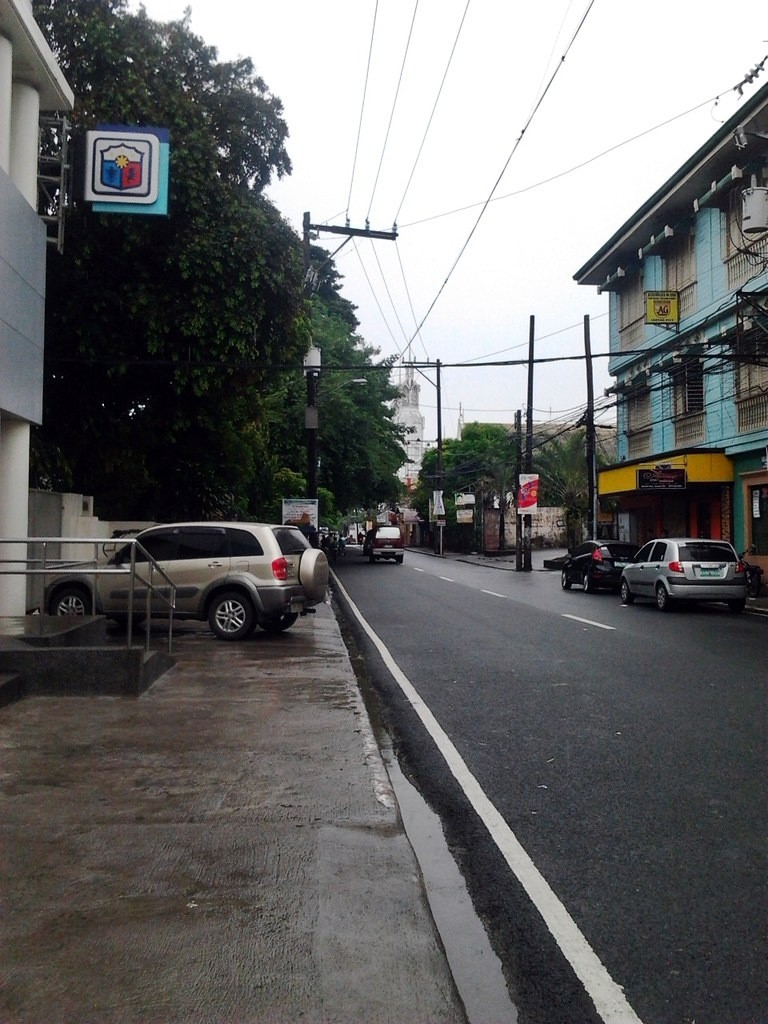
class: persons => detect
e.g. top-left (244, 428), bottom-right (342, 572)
top-left (358, 532), bottom-right (363, 542)
top-left (321, 533), bottom-right (345, 555)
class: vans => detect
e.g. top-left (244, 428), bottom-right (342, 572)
top-left (370, 526), bottom-right (405, 565)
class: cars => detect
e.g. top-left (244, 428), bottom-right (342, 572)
top-left (561, 540), bottom-right (642, 595)
top-left (362, 530), bottom-right (373, 556)
top-left (620, 537), bottom-right (747, 614)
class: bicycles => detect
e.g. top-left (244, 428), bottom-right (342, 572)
top-left (332, 550), bottom-right (338, 564)
top-left (338, 543), bottom-right (346, 557)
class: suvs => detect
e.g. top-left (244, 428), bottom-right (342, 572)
top-left (41, 522), bottom-right (330, 642)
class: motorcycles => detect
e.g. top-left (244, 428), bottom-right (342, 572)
top-left (737, 546), bottom-right (764, 599)
